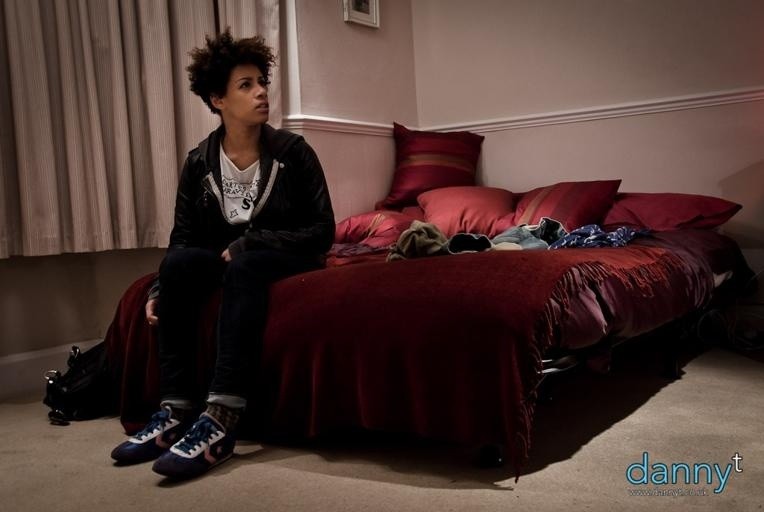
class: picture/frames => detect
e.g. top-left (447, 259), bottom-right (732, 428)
top-left (343, 0), bottom-right (380, 28)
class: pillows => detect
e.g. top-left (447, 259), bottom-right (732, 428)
top-left (602, 192), bottom-right (744, 232)
top-left (418, 185), bottom-right (516, 236)
top-left (372, 120), bottom-right (485, 204)
top-left (513, 179), bottom-right (622, 233)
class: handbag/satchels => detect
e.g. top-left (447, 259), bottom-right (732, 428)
top-left (40, 340), bottom-right (123, 425)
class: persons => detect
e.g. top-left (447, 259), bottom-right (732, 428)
top-left (111, 27), bottom-right (336, 479)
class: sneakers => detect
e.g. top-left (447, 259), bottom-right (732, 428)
top-left (112, 404), bottom-right (185, 464)
top-left (152, 411), bottom-right (236, 478)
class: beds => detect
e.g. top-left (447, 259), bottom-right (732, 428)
top-left (102, 201), bottom-right (751, 486)
